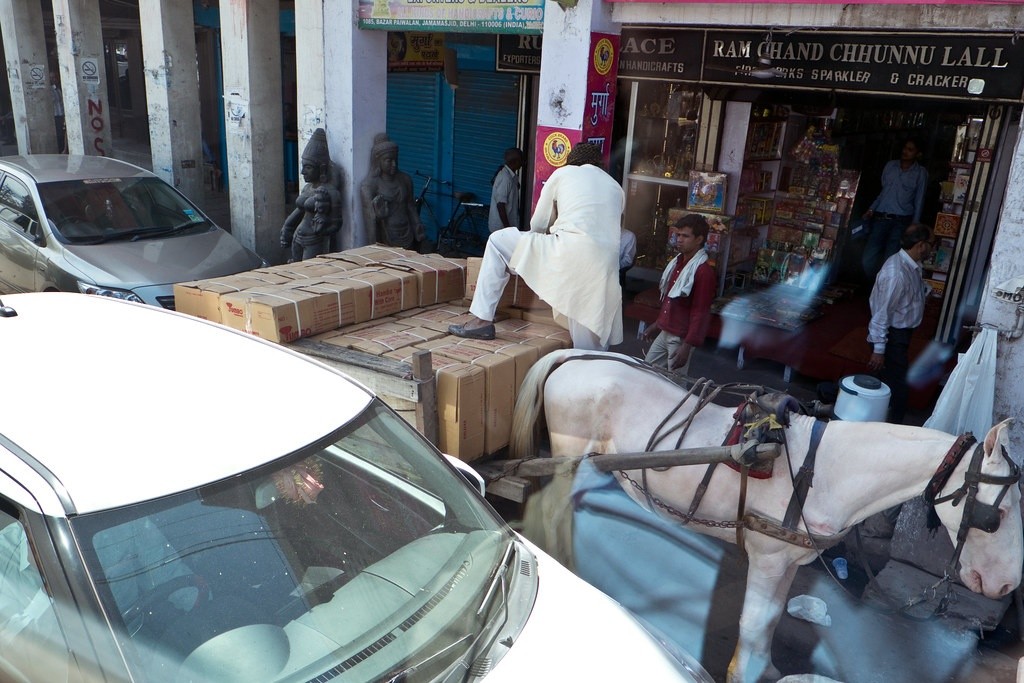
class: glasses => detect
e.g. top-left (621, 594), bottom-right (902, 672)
top-left (925, 240), bottom-right (936, 248)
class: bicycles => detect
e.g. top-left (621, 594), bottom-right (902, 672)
top-left (410, 168), bottom-right (495, 260)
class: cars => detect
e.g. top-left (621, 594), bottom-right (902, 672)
top-left (0, 154), bottom-right (267, 315)
top-left (0, 289), bottom-right (721, 683)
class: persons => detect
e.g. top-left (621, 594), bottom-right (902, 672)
top-left (643, 213), bottom-right (717, 390)
top-left (488, 147), bottom-right (523, 233)
top-left (361, 132), bottom-right (426, 252)
top-left (280, 128), bottom-right (342, 262)
top-left (618, 229), bottom-right (637, 271)
top-left (448, 140), bottom-right (624, 351)
top-left (858, 137), bottom-right (930, 299)
top-left (820, 223), bottom-right (936, 422)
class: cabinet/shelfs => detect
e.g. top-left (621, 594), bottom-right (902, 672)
top-left (618, 80), bottom-right (711, 285)
top-left (719, 99), bottom-right (843, 218)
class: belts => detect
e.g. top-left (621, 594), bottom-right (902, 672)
top-left (877, 212), bottom-right (900, 220)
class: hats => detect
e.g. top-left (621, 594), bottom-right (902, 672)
top-left (562, 141), bottom-right (601, 164)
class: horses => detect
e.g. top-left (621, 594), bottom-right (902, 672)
top-left (504, 348), bottom-right (1024, 682)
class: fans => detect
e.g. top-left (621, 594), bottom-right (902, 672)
top-left (704, 30), bottom-right (812, 79)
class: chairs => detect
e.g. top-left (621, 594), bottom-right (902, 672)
top-left (81, 182), bottom-right (136, 229)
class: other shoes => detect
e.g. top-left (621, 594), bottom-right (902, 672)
top-left (448, 322), bottom-right (496, 340)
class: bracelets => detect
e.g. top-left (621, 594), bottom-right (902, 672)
top-left (869, 209), bottom-right (873, 216)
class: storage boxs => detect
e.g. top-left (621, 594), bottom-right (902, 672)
top-left (919, 116), bottom-right (983, 298)
top-left (172, 240), bottom-right (573, 465)
top-left (666, 122), bottom-right (862, 328)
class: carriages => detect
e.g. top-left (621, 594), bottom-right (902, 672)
top-left (275, 336), bottom-right (1024, 682)
